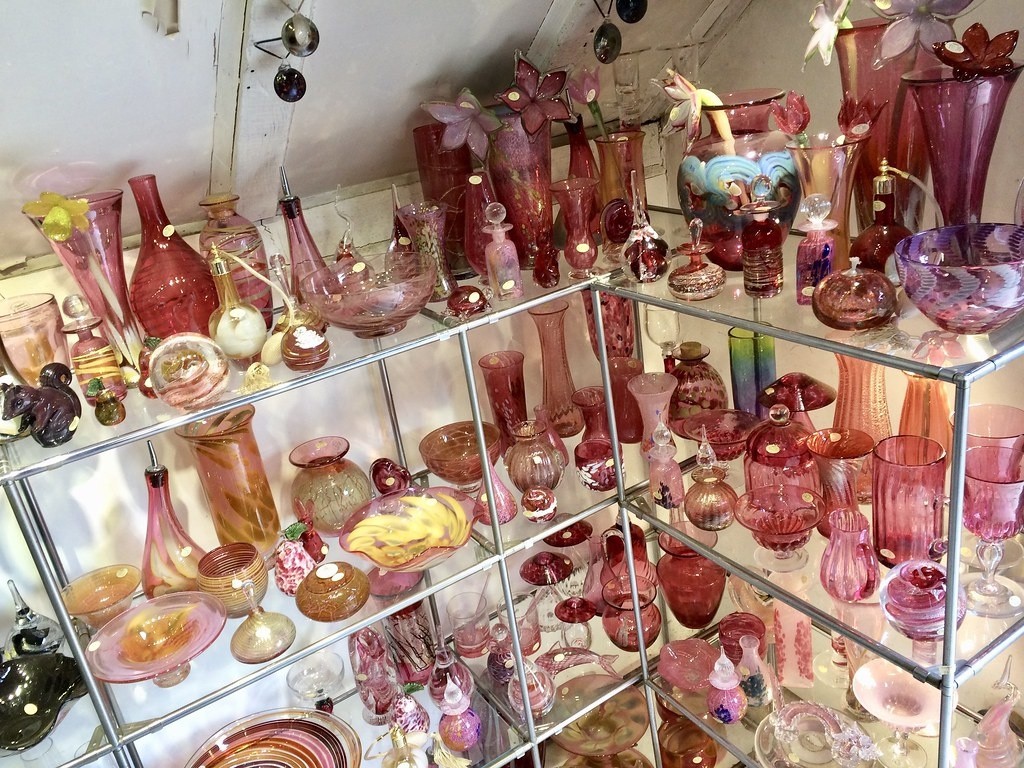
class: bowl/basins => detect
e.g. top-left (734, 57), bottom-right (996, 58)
top-left (418, 421), bottom-right (500, 493)
top-left (184, 707), bottom-right (362, 768)
top-left (296, 253), bottom-right (437, 340)
top-left (894, 222), bottom-right (1024, 336)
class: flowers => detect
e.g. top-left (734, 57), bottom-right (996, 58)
top-left (564, 63), bottom-right (608, 142)
top-left (802, 0), bottom-right (853, 72)
top-left (420, 86), bottom-right (508, 161)
top-left (768, 92), bottom-right (811, 148)
top-left (651, 68), bottom-right (734, 151)
top-left (20, 195), bottom-right (91, 243)
top-left (495, 48), bottom-right (579, 142)
top-left (863, 0), bottom-right (984, 70)
top-left (932, 25), bottom-right (1019, 82)
top-left (837, 86), bottom-right (890, 142)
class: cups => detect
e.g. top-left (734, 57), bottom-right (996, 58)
top-left (0, 0), bottom-right (1024, 767)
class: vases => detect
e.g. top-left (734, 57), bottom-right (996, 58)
top-left (2, 0), bottom-right (1024, 707)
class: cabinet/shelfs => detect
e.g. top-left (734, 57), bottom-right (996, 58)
top-left (0, 200), bottom-right (1022, 768)
